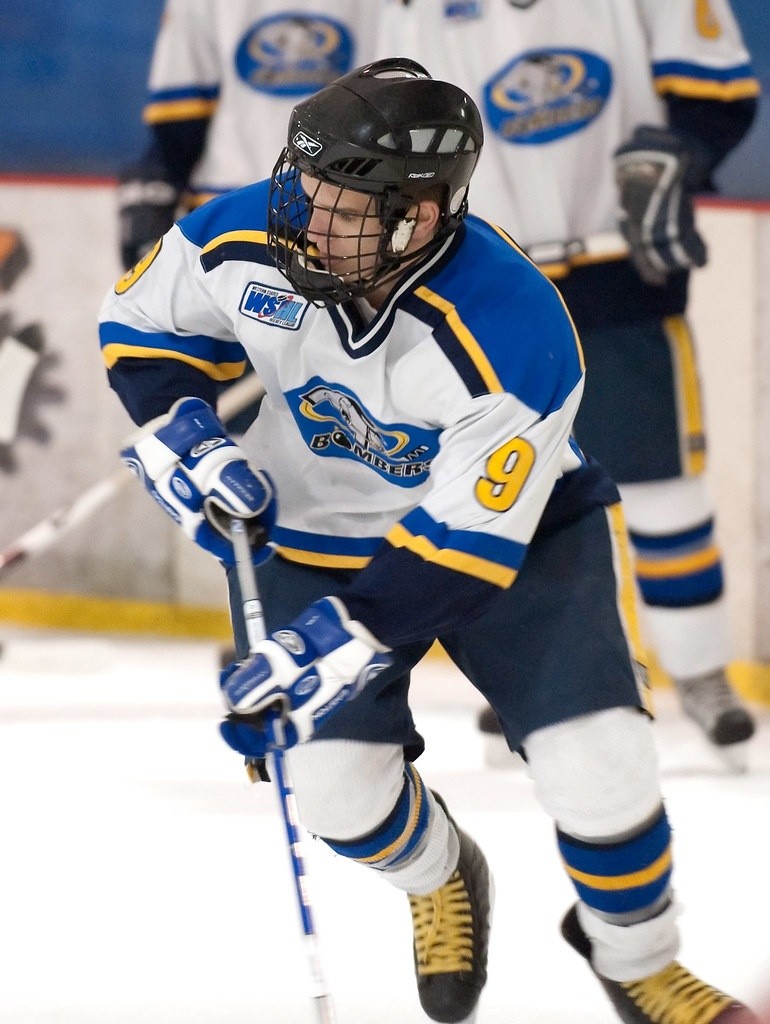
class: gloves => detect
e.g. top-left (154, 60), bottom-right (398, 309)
top-left (613, 126), bottom-right (706, 281)
top-left (117, 167), bottom-right (179, 270)
top-left (116, 396), bottom-right (277, 568)
top-left (218, 595), bottom-right (395, 754)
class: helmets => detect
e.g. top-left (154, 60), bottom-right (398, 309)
top-left (266, 58), bottom-right (483, 310)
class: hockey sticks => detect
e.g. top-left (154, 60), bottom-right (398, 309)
top-left (521, 227), bottom-right (628, 265)
top-left (230, 517), bottom-right (339, 1024)
top-left (0, 372), bottom-right (266, 575)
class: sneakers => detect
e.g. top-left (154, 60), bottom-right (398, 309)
top-left (406, 788), bottom-right (492, 1024)
top-left (559, 900), bottom-right (761, 1024)
top-left (669, 667), bottom-right (757, 776)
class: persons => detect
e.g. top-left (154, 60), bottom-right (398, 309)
top-left (122, 1), bottom-right (759, 753)
top-left (99, 59), bottom-right (762, 1024)
